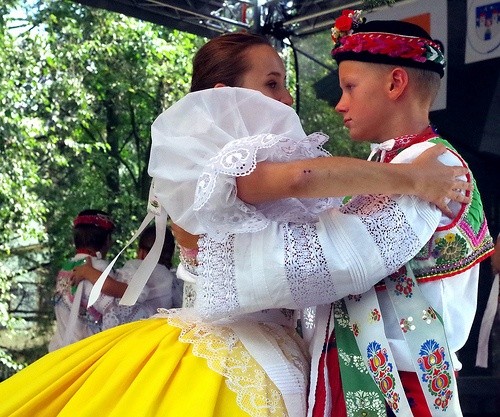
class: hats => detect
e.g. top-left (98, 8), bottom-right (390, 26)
top-left (74, 208), bottom-right (114, 232)
top-left (331, 10), bottom-right (446, 79)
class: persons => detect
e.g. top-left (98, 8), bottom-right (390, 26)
top-left (0, 32), bottom-right (475, 417)
top-left (70, 224), bottom-right (183, 330)
top-left (47, 209), bottom-right (118, 353)
top-left (169, 20), bottom-right (496, 417)
top-left (475, 230), bottom-right (500, 274)
